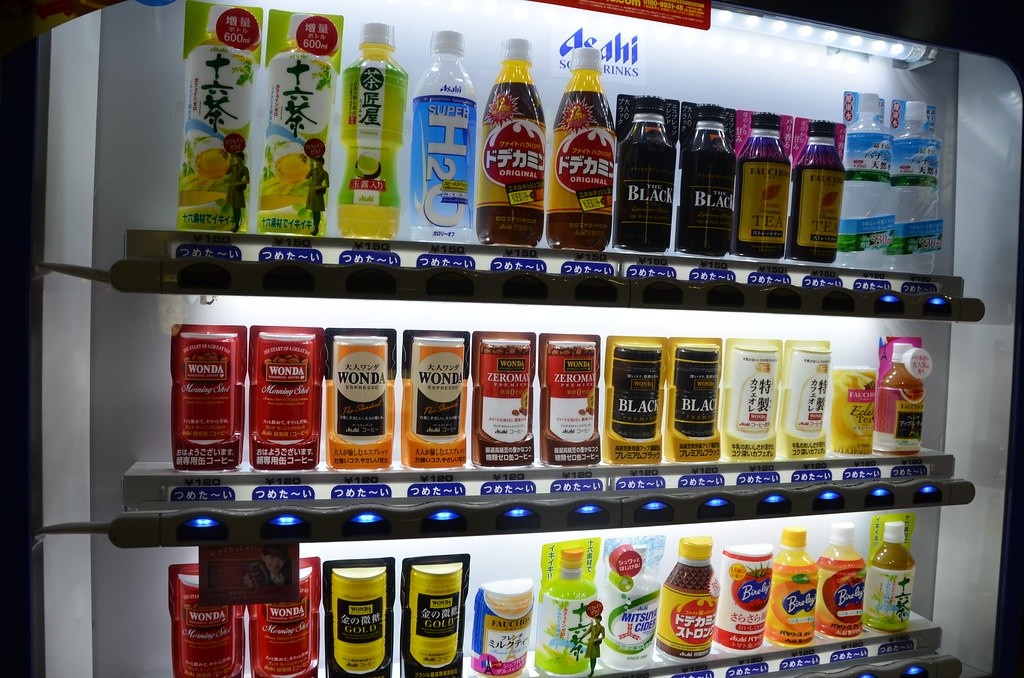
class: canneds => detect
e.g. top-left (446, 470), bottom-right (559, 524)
top-left (173, 332), bottom-right (878, 678)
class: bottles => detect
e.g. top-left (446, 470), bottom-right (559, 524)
top-left (655, 536), bottom-right (721, 660)
top-left (474, 37), bottom-right (546, 246)
top-left (763, 526), bottom-right (820, 647)
top-left (786, 119), bottom-right (845, 265)
top-left (675, 104), bottom-right (736, 258)
top-left (600, 544), bottom-right (661, 671)
top-left (409, 29), bottom-right (478, 245)
top-left (837, 92), bottom-right (895, 272)
top-left (614, 95), bottom-right (678, 254)
top-left (548, 46), bottom-right (617, 252)
top-left (534, 548), bottom-right (597, 675)
top-left (890, 101), bottom-right (944, 274)
top-left (733, 111), bottom-right (790, 260)
top-left (178, 5), bottom-right (260, 234)
top-left (863, 521), bottom-right (915, 632)
top-left (873, 342), bottom-right (925, 456)
top-left (257, 12), bottom-right (338, 237)
top-left (815, 523), bottom-right (867, 641)
top-left (336, 21), bottom-right (410, 240)
